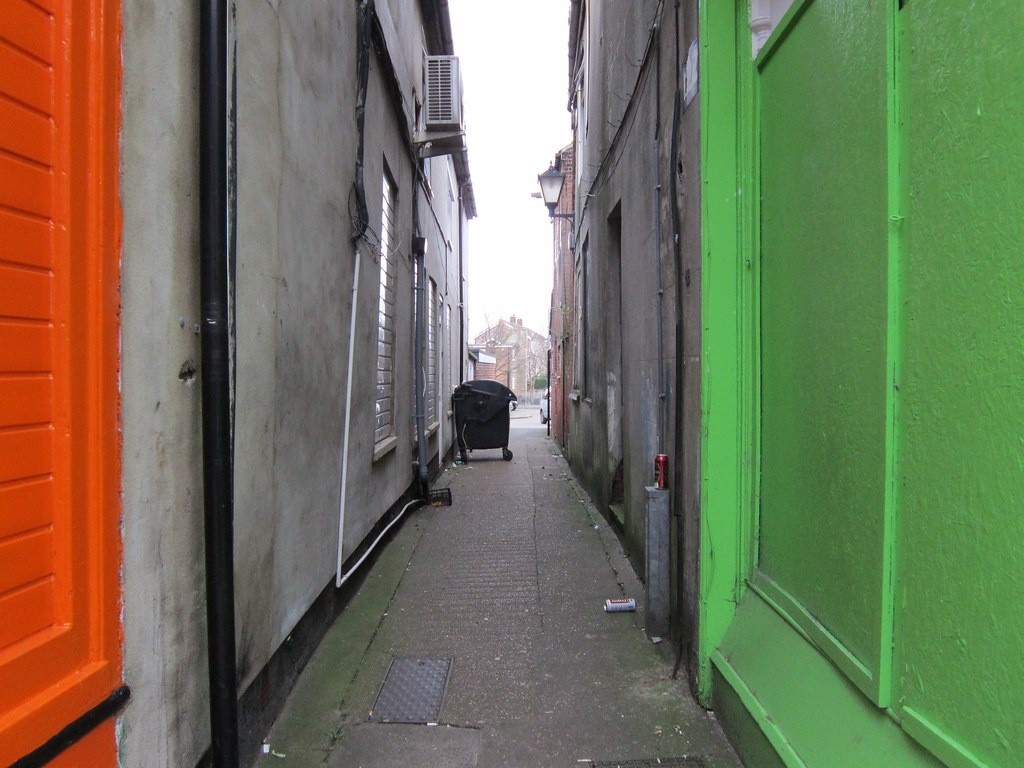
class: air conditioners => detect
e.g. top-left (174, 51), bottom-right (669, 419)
top-left (424, 55), bottom-right (464, 148)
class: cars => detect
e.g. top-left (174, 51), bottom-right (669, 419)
top-left (540, 386), bottom-right (552, 424)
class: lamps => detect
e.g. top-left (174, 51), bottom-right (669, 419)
top-left (537, 160), bottom-right (575, 228)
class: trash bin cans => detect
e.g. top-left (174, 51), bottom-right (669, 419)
top-left (451, 380), bottom-right (514, 460)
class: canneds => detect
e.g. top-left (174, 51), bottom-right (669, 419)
top-left (654, 454), bottom-right (669, 490)
